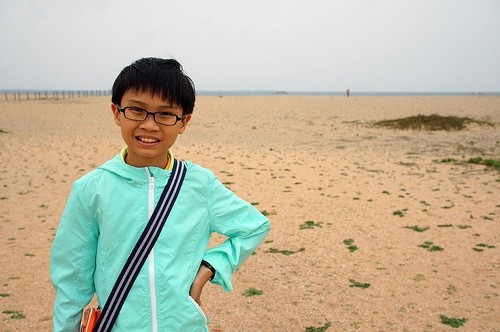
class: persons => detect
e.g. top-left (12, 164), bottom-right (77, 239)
top-left (48, 57), bottom-right (271, 332)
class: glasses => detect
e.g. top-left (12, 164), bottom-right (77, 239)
top-left (116, 105), bottom-right (186, 125)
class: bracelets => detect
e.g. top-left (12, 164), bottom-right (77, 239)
top-left (200, 260), bottom-right (216, 281)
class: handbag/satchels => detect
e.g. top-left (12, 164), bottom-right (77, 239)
top-left (80, 307), bottom-right (113, 332)
